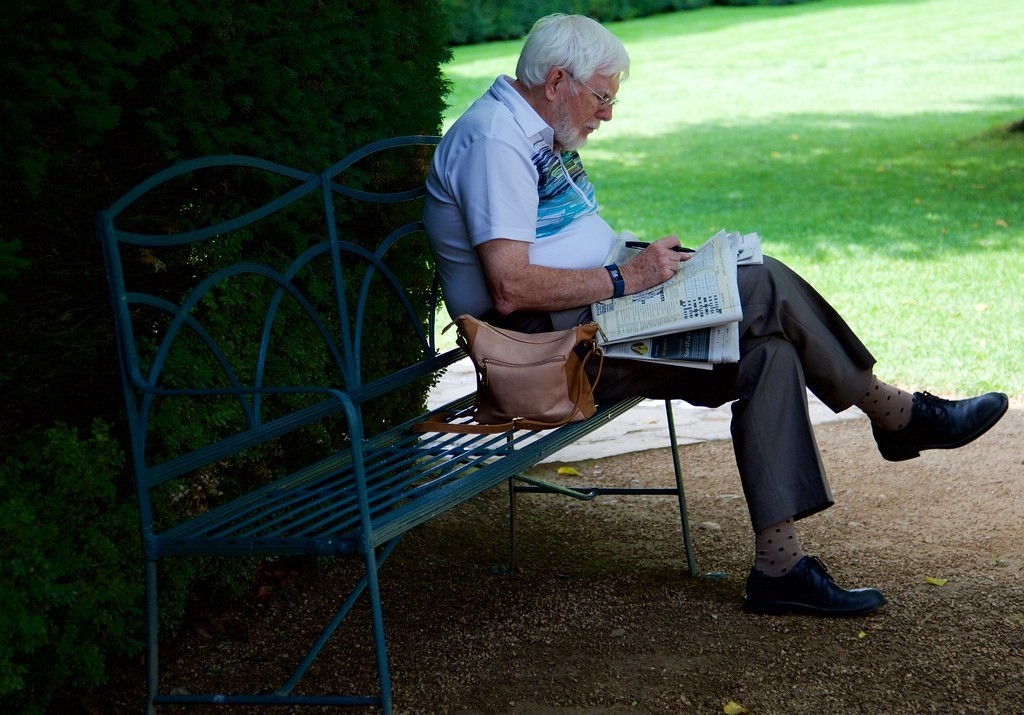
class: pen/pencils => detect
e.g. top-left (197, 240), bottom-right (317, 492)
top-left (623, 240), bottom-right (699, 255)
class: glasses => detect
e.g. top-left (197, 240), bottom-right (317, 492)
top-left (565, 69), bottom-right (619, 110)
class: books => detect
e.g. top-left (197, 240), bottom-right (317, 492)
top-left (590, 228), bottom-right (764, 369)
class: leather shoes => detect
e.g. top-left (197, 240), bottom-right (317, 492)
top-left (744, 555), bottom-right (887, 619)
top-left (871, 391), bottom-right (1009, 462)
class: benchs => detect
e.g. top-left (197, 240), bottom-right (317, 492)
top-left (104, 134), bottom-right (726, 715)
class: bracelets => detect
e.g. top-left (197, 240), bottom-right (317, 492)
top-left (604, 263), bottom-right (625, 298)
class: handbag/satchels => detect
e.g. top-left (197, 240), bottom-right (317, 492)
top-left (409, 314), bottom-right (604, 435)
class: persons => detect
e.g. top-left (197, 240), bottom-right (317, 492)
top-left (427, 14), bottom-right (1009, 619)
